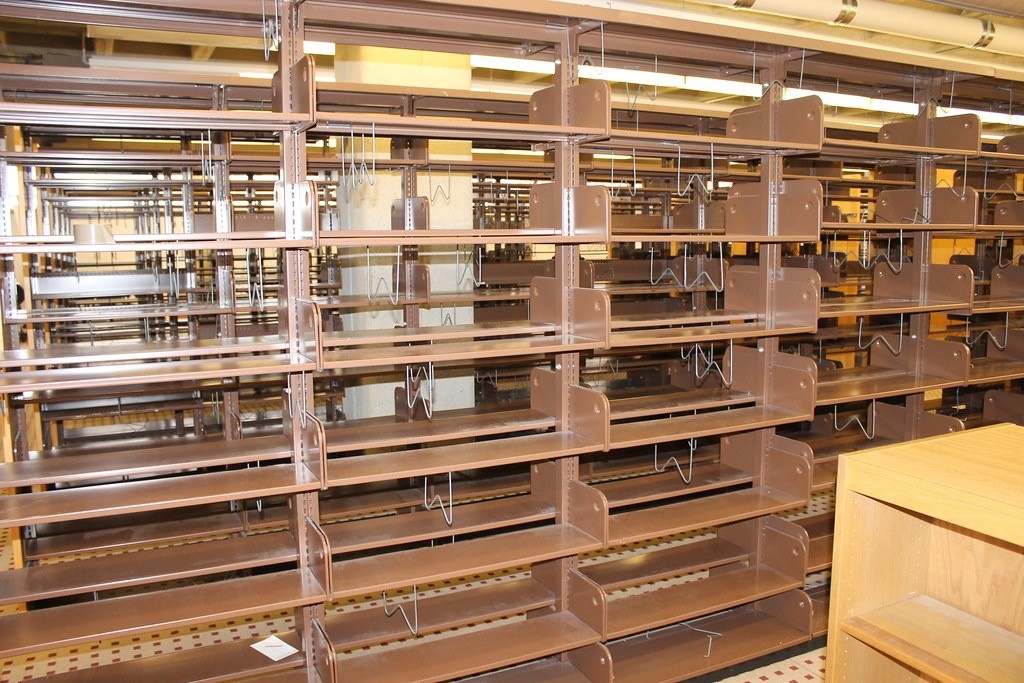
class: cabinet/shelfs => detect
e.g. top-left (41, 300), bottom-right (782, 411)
top-left (825, 422), bottom-right (1024, 683)
